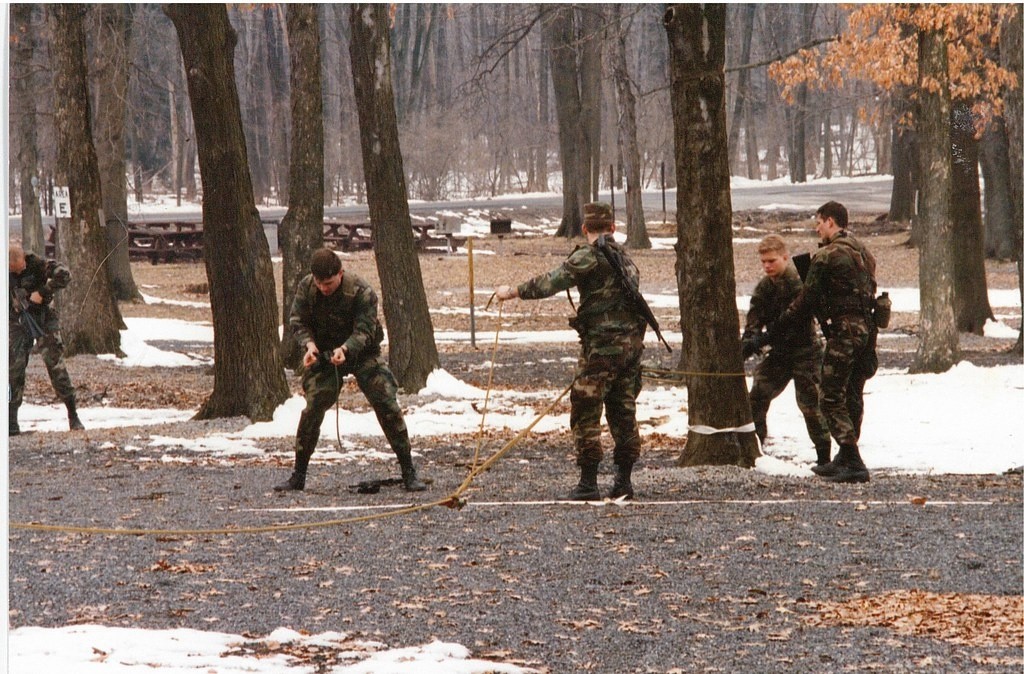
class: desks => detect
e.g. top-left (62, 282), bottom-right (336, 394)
top-left (129, 230), bottom-right (203, 248)
top-left (324, 220), bottom-right (435, 238)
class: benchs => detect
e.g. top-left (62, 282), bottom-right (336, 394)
top-left (44, 242), bottom-right (205, 265)
top-left (325, 233), bottom-right (467, 252)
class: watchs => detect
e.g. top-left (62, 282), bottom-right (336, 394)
top-left (508, 287), bottom-right (515, 297)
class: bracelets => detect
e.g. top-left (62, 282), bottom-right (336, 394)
top-left (341, 346), bottom-right (345, 352)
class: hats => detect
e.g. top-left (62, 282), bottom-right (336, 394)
top-left (584, 202), bottom-right (613, 223)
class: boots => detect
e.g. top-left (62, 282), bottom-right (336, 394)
top-left (557, 463), bottom-right (600, 500)
top-left (603, 462), bottom-right (634, 500)
top-left (817, 448), bottom-right (830, 466)
top-left (397, 454), bottom-right (426, 491)
top-left (811, 443), bottom-right (870, 483)
top-left (9, 406), bottom-right (20, 435)
top-left (273, 454), bottom-right (310, 491)
top-left (65, 399), bottom-right (85, 430)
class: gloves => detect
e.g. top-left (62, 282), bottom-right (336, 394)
top-left (759, 331), bottom-right (769, 347)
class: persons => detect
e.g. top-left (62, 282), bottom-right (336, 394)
top-left (273, 249), bottom-right (427, 492)
top-left (495, 201), bottom-right (648, 501)
top-left (741, 233), bottom-right (832, 466)
top-left (774, 200), bottom-right (878, 484)
top-left (9, 239), bottom-right (85, 436)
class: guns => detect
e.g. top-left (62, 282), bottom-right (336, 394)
top-left (598, 232), bottom-right (674, 353)
top-left (15, 286), bottom-right (48, 339)
top-left (792, 252), bottom-right (831, 340)
